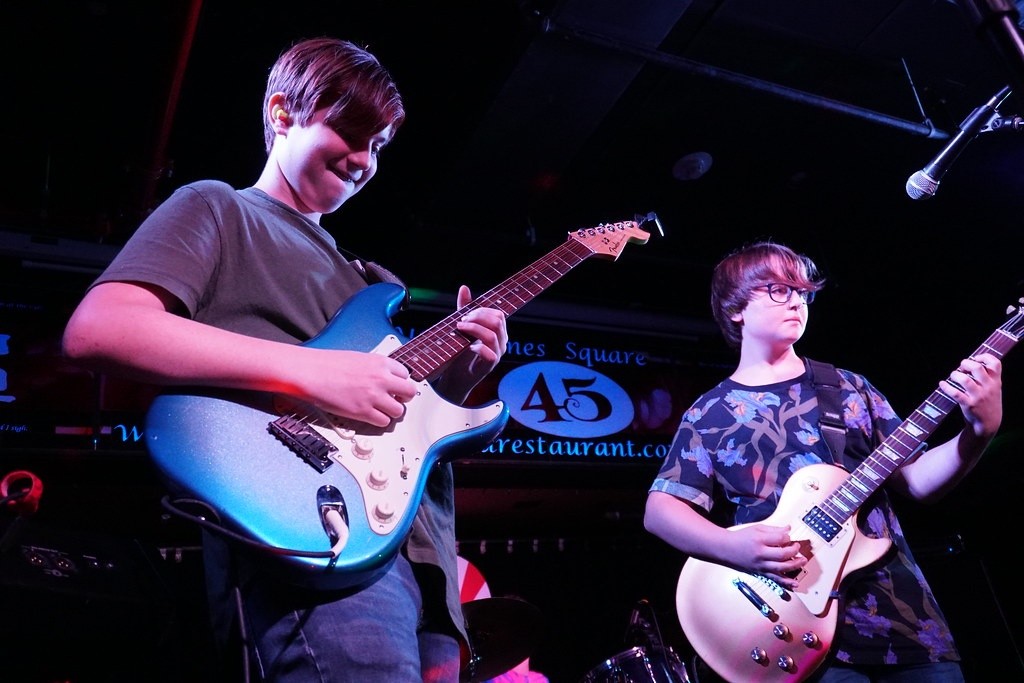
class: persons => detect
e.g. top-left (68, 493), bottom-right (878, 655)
top-left (63, 39), bottom-right (508, 683)
top-left (644, 244), bottom-right (1002, 683)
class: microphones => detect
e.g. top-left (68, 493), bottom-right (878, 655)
top-left (906, 88), bottom-right (1010, 200)
top-left (624, 601), bottom-right (642, 647)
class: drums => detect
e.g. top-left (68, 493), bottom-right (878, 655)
top-left (583, 644), bottom-right (691, 683)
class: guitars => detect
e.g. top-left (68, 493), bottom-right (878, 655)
top-left (132, 217), bottom-right (655, 595)
top-left (672, 292), bottom-right (1024, 683)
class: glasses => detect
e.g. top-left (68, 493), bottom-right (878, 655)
top-left (747, 282), bottom-right (815, 306)
